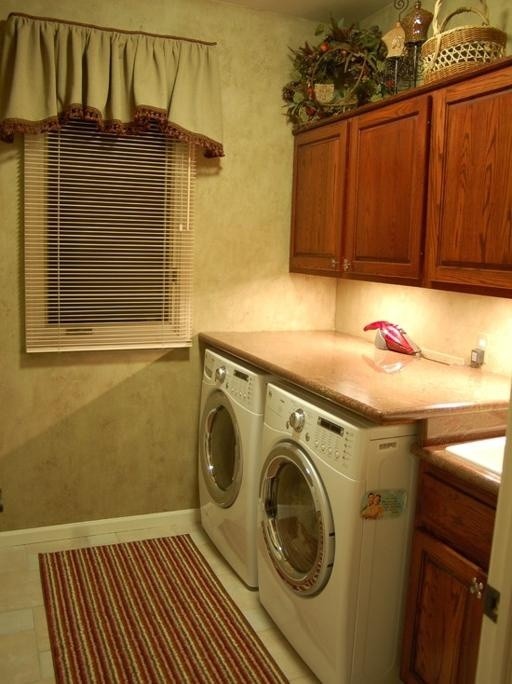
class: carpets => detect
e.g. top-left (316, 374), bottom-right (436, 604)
top-left (38, 534), bottom-right (290, 683)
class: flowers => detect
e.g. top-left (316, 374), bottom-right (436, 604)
top-left (282, 23), bottom-right (411, 128)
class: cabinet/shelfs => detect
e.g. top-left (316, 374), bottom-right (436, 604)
top-left (427, 65), bottom-right (512, 300)
top-left (290, 94), bottom-right (430, 286)
top-left (398, 457), bottom-right (501, 684)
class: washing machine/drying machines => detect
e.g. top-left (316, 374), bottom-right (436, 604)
top-left (255, 379), bottom-right (419, 683)
top-left (198, 348), bottom-right (264, 590)
